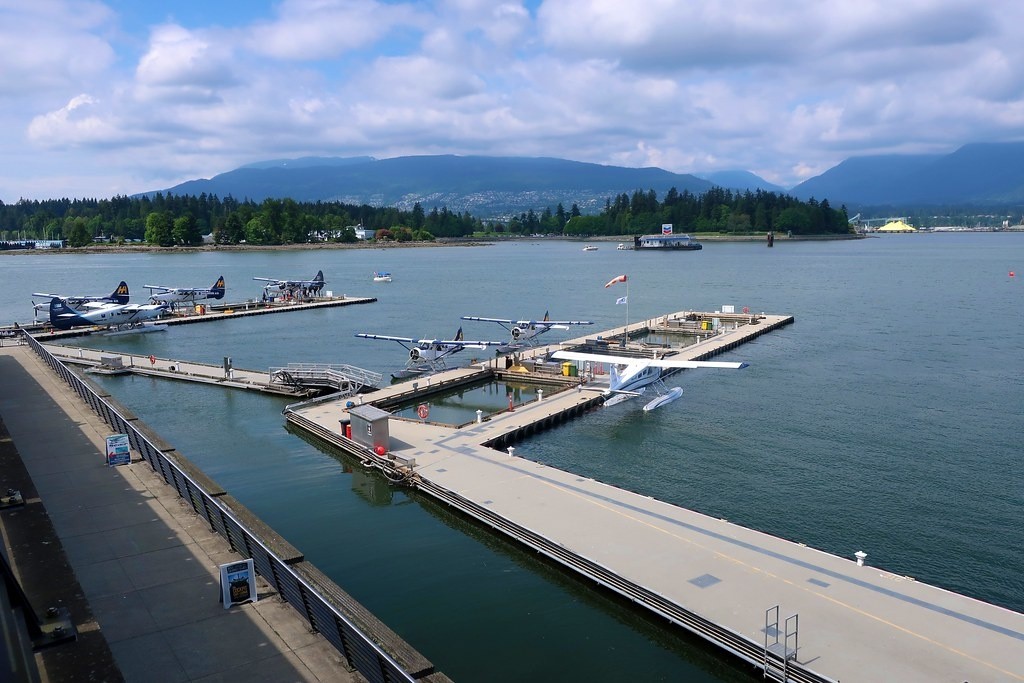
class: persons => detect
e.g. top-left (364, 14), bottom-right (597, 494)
top-left (262, 289), bottom-right (268, 302)
top-left (170, 301), bottom-right (175, 313)
top-left (294, 287), bottom-right (302, 305)
top-left (14, 322), bottom-right (19, 329)
top-left (289, 287), bottom-right (293, 297)
top-left (153, 300), bottom-right (167, 305)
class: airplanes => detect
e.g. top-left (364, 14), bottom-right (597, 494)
top-left (460, 310), bottom-right (594, 353)
top-left (143, 276), bottom-right (226, 302)
top-left (252, 270), bottom-right (327, 298)
top-left (31, 281), bottom-right (170, 336)
top-left (354, 326), bottom-right (508, 379)
top-left (551, 351), bottom-right (751, 411)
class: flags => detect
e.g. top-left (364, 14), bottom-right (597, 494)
top-left (605, 275), bottom-right (627, 288)
top-left (616, 297), bottom-right (628, 304)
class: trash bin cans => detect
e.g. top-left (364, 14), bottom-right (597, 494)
top-left (562, 362), bottom-right (578, 377)
top-left (701, 320), bottom-right (712, 330)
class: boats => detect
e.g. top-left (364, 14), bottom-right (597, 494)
top-left (582, 246), bottom-right (599, 251)
top-left (373, 272), bottom-right (392, 282)
top-left (617, 234), bottom-right (702, 250)
